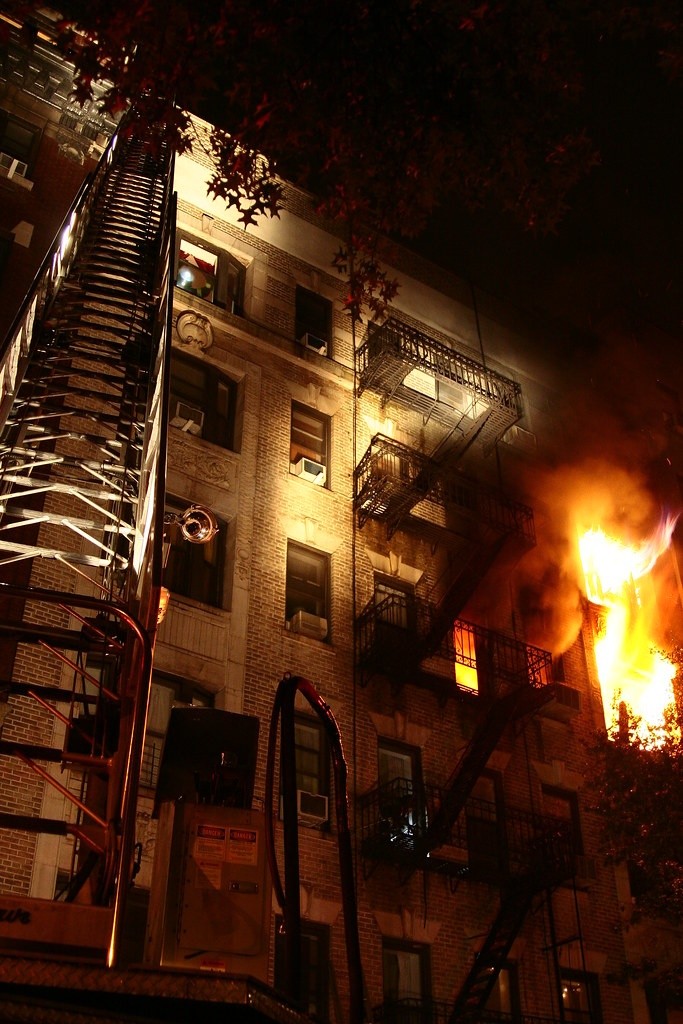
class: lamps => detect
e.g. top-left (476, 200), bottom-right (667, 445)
top-left (164, 503), bottom-right (219, 544)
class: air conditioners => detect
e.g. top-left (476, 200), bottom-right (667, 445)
top-left (169, 401), bottom-right (204, 435)
top-left (291, 610), bottom-right (327, 640)
top-left (296, 457), bottom-right (326, 486)
top-left (0, 152), bottom-right (27, 177)
top-left (280, 790), bottom-right (328, 827)
top-left (299, 332), bottom-right (328, 356)
